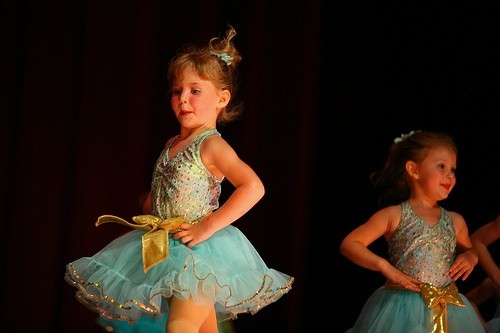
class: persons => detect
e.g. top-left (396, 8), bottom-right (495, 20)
top-left (63, 24), bottom-right (295, 333)
top-left (338, 130), bottom-right (488, 333)
top-left (466, 216), bottom-right (500, 333)
